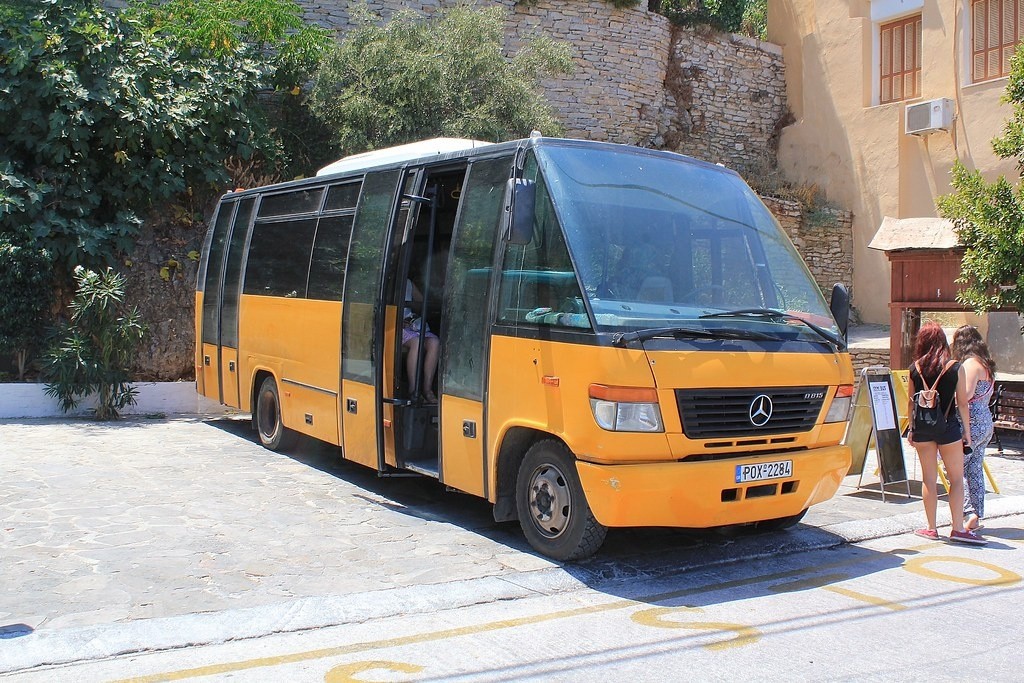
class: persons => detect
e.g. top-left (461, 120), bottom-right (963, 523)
top-left (950, 324), bottom-right (999, 532)
top-left (907, 322), bottom-right (989, 544)
top-left (402, 279), bottom-right (438, 403)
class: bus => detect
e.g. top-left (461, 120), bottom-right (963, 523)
top-left (191, 127), bottom-right (857, 565)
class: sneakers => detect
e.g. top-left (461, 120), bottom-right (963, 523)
top-left (913, 528), bottom-right (939, 540)
top-left (949, 530), bottom-right (989, 547)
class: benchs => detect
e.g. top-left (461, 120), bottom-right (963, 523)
top-left (988, 385), bottom-right (1024, 451)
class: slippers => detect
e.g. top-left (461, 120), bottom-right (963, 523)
top-left (966, 525), bottom-right (984, 532)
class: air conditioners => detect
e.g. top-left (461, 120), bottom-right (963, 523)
top-left (904, 97), bottom-right (955, 135)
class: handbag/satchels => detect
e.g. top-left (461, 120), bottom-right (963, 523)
top-left (409, 313), bottom-right (430, 332)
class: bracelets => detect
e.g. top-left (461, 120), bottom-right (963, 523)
top-left (907, 428), bottom-right (913, 432)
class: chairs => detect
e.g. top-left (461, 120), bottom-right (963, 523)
top-left (606, 242), bottom-right (673, 302)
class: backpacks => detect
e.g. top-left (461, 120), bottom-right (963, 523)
top-left (909, 359), bottom-right (958, 435)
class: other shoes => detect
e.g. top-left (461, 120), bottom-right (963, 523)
top-left (408, 393), bottom-right (422, 408)
top-left (420, 391), bottom-right (438, 406)
